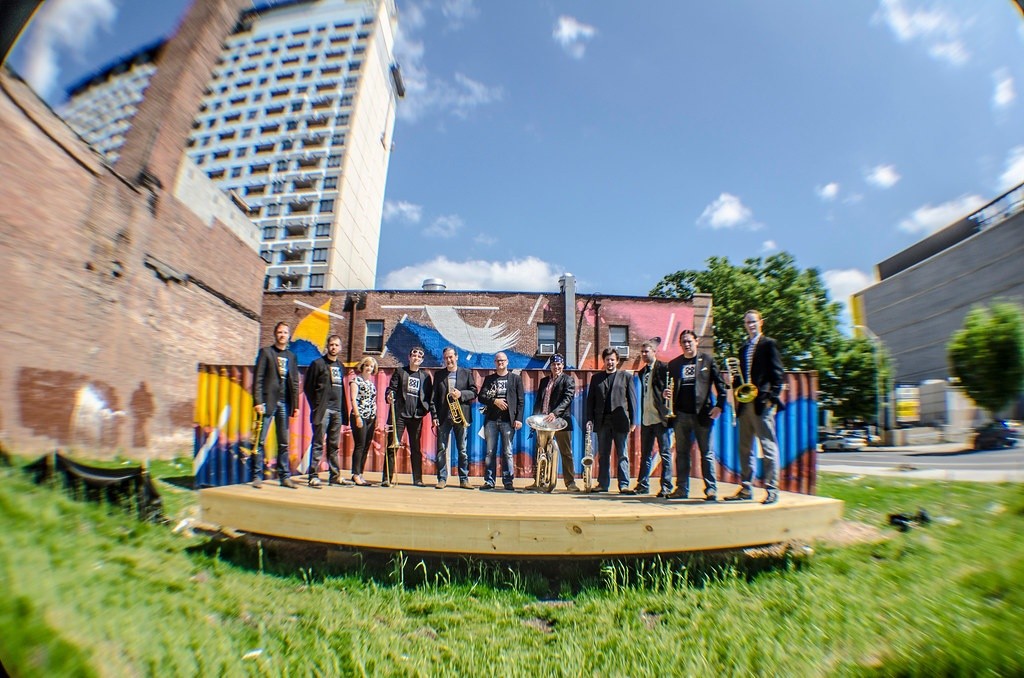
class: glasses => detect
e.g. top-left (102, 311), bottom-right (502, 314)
top-left (412, 349), bottom-right (425, 356)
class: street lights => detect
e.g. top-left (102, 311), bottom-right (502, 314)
top-left (848, 325), bottom-right (878, 437)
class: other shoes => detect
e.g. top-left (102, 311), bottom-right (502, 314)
top-left (505, 484), bottom-right (515, 491)
top-left (633, 488), bottom-right (649, 494)
top-left (591, 485), bottom-right (608, 492)
top-left (254, 478), bottom-right (261, 490)
top-left (763, 494), bottom-right (779, 504)
top-left (566, 484), bottom-right (580, 491)
top-left (723, 494), bottom-right (753, 501)
top-left (381, 482), bottom-right (393, 487)
top-left (480, 483), bottom-right (495, 490)
top-left (436, 481), bottom-right (445, 488)
top-left (414, 480), bottom-right (426, 487)
top-left (621, 488), bottom-right (635, 494)
top-left (310, 479), bottom-right (323, 489)
top-left (280, 478), bottom-right (298, 489)
top-left (666, 492), bottom-right (688, 499)
top-left (330, 480), bottom-right (355, 488)
top-left (707, 492), bottom-right (717, 500)
top-left (526, 484), bottom-right (536, 490)
top-left (460, 483), bottom-right (474, 490)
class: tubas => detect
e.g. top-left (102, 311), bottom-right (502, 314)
top-left (525, 414), bottom-right (569, 493)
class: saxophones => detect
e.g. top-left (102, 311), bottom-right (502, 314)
top-left (580, 424), bottom-right (595, 493)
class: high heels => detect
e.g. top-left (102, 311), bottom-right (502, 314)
top-left (351, 476), bottom-right (372, 486)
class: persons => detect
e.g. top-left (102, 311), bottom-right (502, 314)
top-left (586, 347), bottom-right (636, 495)
top-left (661, 330), bottom-right (727, 501)
top-left (379, 344), bottom-right (432, 487)
top-left (303, 335), bottom-right (349, 485)
top-left (631, 340), bottom-right (674, 498)
top-left (252, 321), bottom-right (300, 489)
top-left (724, 310), bottom-right (784, 504)
top-left (479, 351), bottom-right (524, 490)
top-left (429, 348), bottom-right (478, 489)
top-left (349, 357), bottom-right (377, 486)
top-left (525, 353), bottom-right (579, 492)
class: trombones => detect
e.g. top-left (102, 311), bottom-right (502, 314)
top-left (382, 389), bottom-right (406, 489)
top-left (725, 356), bottom-right (758, 427)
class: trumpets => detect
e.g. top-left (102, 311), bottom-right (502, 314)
top-left (663, 371), bottom-right (677, 419)
top-left (446, 386), bottom-right (472, 429)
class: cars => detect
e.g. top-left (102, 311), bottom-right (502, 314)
top-left (974, 418), bottom-right (1024, 451)
top-left (821, 435), bottom-right (869, 453)
